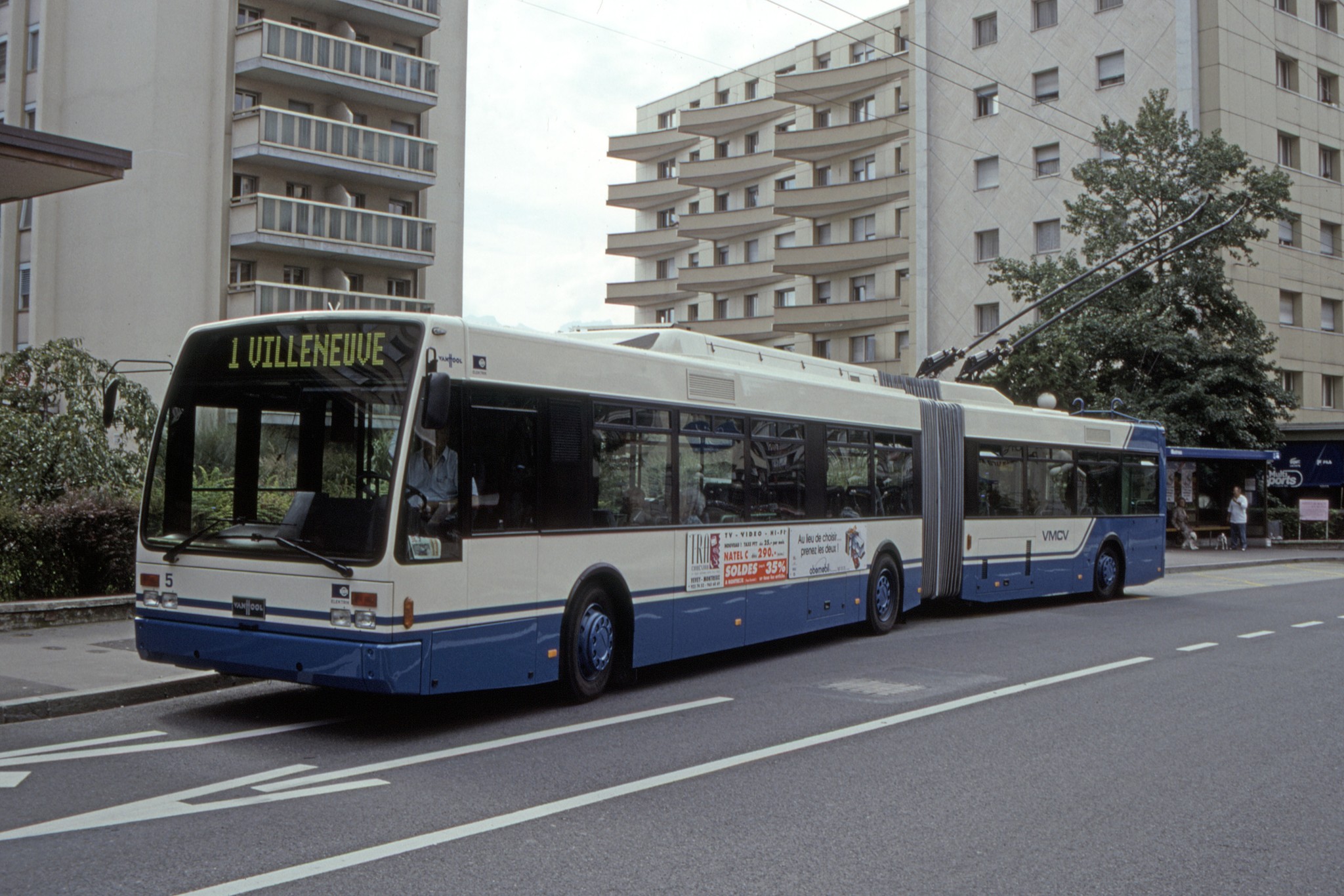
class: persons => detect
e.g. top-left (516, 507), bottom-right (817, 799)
top-left (1171, 497), bottom-right (1198, 550)
top-left (404, 418), bottom-right (481, 527)
top-left (624, 487), bottom-right (653, 525)
top-left (1226, 487), bottom-right (1249, 551)
top-left (679, 487), bottom-right (706, 525)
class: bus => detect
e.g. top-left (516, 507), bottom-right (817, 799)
top-left (99, 190), bottom-right (1252, 704)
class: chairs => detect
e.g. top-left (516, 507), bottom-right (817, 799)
top-left (702, 482), bottom-right (901, 520)
top-left (471, 494), bottom-right (500, 525)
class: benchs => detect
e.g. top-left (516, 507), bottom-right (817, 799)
top-left (1166, 525), bottom-right (1230, 546)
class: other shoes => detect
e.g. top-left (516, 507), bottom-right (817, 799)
top-left (1182, 543), bottom-right (1187, 549)
top-left (1230, 546), bottom-right (1236, 550)
top-left (1191, 546), bottom-right (1199, 550)
top-left (1242, 547), bottom-right (1246, 551)
